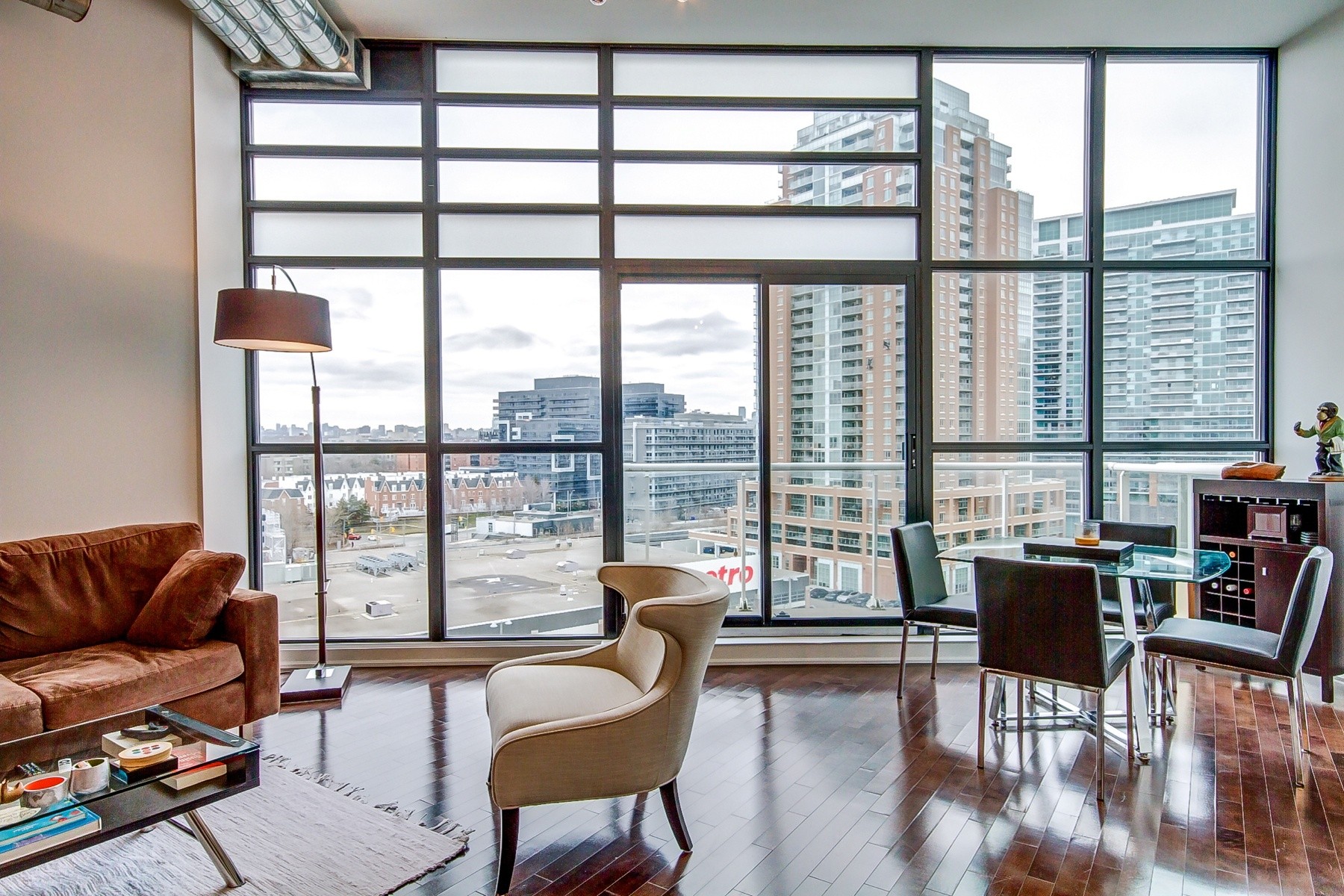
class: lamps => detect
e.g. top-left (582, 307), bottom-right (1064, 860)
top-left (214, 264), bottom-right (353, 704)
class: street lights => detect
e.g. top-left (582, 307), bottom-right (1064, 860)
top-left (570, 489), bottom-right (576, 511)
top-left (342, 512), bottom-right (357, 549)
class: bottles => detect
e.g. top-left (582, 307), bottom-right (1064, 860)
top-left (1211, 582), bottom-right (1221, 589)
top-left (1228, 551), bottom-right (1238, 559)
top-left (1243, 588), bottom-right (1255, 594)
top-left (1226, 584), bottom-right (1238, 592)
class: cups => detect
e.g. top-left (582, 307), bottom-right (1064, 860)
top-left (1074, 522), bottom-right (1101, 545)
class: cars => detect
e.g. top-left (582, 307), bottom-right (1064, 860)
top-left (885, 600), bottom-right (900, 608)
top-left (836, 591), bottom-right (860, 604)
top-left (367, 535), bottom-right (383, 541)
top-left (824, 590), bottom-right (844, 602)
top-left (853, 593), bottom-right (872, 606)
top-left (348, 533), bottom-right (361, 540)
top-left (810, 588), bottom-right (828, 598)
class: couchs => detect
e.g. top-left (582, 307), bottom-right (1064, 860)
top-left (0, 522), bottom-right (280, 777)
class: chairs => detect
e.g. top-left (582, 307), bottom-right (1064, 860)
top-left (1052, 520), bottom-right (1178, 701)
top-left (973, 556), bottom-right (1135, 799)
top-left (1141, 546), bottom-right (1335, 787)
top-left (890, 520), bottom-right (978, 699)
top-left (485, 562), bottom-right (730, 896)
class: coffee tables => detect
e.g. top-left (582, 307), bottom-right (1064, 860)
top-left (0, 705), bottom-right (260, 888)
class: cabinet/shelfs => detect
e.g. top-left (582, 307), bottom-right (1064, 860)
top-left (1191, 477), bottom-right (1344, 702)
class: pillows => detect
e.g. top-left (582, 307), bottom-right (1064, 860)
top-left (125, 550), bottom-right (246, 648)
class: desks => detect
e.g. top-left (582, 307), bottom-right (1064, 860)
top-left (933, 536), bottom-right (1232, 762)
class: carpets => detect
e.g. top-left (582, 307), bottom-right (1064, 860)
top-left (0, 749), bottom-right (476, 896)
